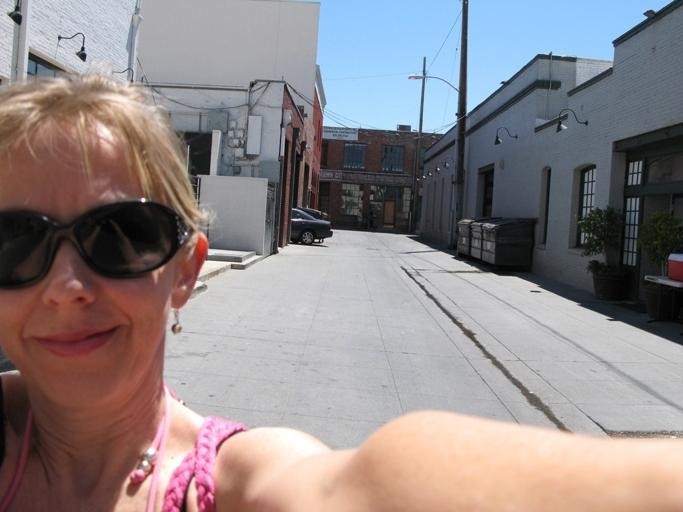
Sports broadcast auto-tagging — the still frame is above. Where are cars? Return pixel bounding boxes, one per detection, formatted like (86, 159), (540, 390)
(290, 208), (332, 244)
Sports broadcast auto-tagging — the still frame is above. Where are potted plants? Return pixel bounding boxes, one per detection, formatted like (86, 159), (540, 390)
(574, 205), (683, 321)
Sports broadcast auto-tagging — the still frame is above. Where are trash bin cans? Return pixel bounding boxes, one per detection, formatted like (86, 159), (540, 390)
(455, 215), (538, 268)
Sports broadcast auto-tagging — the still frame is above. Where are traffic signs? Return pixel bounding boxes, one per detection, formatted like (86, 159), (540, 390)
(407, 75), (458, 248)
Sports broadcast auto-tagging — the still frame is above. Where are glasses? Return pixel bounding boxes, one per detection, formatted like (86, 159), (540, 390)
(1, 199), (190, 290)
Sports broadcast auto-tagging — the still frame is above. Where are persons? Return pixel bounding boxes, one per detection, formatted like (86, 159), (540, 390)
(0, 72), (683, 512)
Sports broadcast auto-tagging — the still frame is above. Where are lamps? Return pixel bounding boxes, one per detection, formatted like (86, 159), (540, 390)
(556, 108), (588, 132)
(494, 126), (518, 145)
(59, 32), (87, 62)
(8, 0), (22, 25)
